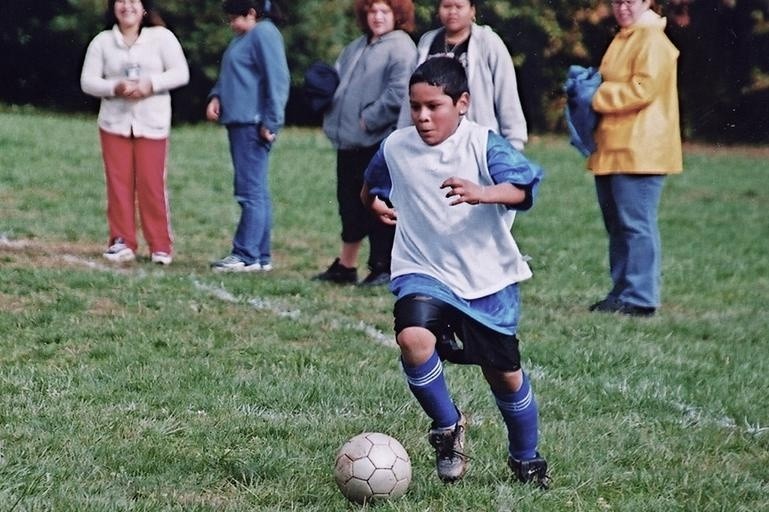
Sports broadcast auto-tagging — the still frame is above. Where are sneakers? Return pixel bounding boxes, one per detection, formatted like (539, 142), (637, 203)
(311, 257), (358, 284)
(507, 454), (551, 490)
(257, 262), (271, 271)
(357, 268), (391, 287)
(151, 251), (172, 265)
(103, 240), (135, 262)
(591, 297), (615, 310)
(428, 406), (467, 480)
(604, 300), (654, 316)
(212, 255), (261, 273)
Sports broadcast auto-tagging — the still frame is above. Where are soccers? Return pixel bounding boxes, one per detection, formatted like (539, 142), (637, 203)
(333, 432), (412, 507)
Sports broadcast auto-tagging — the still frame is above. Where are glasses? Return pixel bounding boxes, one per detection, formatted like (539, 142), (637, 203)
(610, 0), (638, 6)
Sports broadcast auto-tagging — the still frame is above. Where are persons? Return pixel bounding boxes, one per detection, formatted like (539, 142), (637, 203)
(391, 0), (527, 150)
(311, 1), (420, 288)
(79, 0), (190, 261)
(360, 55), (555, 485)
(206, 1), (291, 271)
(578, 0), (680, 314)
